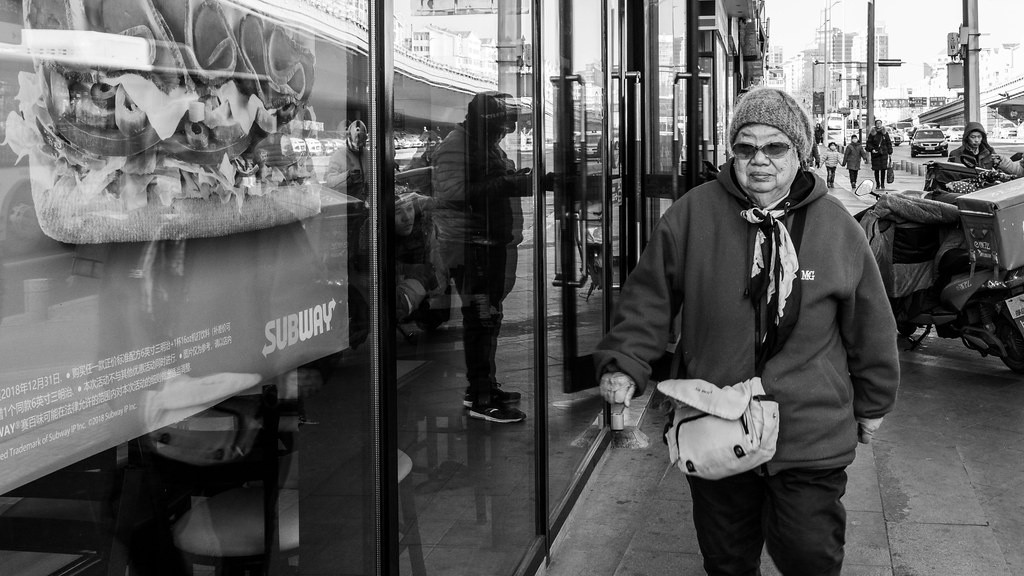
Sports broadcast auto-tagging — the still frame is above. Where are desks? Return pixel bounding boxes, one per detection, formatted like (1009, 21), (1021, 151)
(396, 359), (437, 575)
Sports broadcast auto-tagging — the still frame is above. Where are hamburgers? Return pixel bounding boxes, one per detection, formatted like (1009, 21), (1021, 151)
(4, 1), (322, 242)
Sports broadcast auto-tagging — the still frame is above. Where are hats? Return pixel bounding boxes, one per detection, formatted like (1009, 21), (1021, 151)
(730, 88), (813, 168)
(470, 94), (518, 123)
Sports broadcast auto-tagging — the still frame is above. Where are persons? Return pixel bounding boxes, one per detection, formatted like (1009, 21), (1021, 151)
(948, 122), (997, 170)
(816, 124), (825, 146)
(595, 88), (900, 576)
(807, 142), (820, 168)
(325, 91), (565, 421)
(842, 135), (868, 188)
(706, 165), (723, 180)
(820, 142), (843, 188)
(991, 154), (1024, 177)
(865, 120), (893, 189)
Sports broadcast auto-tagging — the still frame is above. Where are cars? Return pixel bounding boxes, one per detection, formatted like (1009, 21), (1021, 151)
(909, 128), (951, 157)
(846, 122), (966, 145)
(573, 130), (602, 159)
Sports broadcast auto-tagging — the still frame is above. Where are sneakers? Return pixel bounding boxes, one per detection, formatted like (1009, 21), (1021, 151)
(469, 402), (526, 423)
(461, 386), (521, 409)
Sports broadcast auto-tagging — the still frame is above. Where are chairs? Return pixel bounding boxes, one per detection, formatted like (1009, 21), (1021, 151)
(0, 388), (413, 576)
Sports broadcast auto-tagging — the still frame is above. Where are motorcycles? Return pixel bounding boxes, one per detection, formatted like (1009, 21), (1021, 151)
(850, 179), (1024, 376)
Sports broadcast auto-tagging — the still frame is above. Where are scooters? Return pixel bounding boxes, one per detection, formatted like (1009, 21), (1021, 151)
(569, 208), (605, 301)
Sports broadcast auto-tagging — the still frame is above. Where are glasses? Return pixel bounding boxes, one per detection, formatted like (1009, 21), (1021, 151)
(490, 124), (516, 136)
(731, 142), (795, 159)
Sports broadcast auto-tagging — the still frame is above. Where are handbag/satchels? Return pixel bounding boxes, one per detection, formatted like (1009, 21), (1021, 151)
(872, 147), (883, 159)
(656, 377), (780, 479)
(887, 157), (894, 184)
